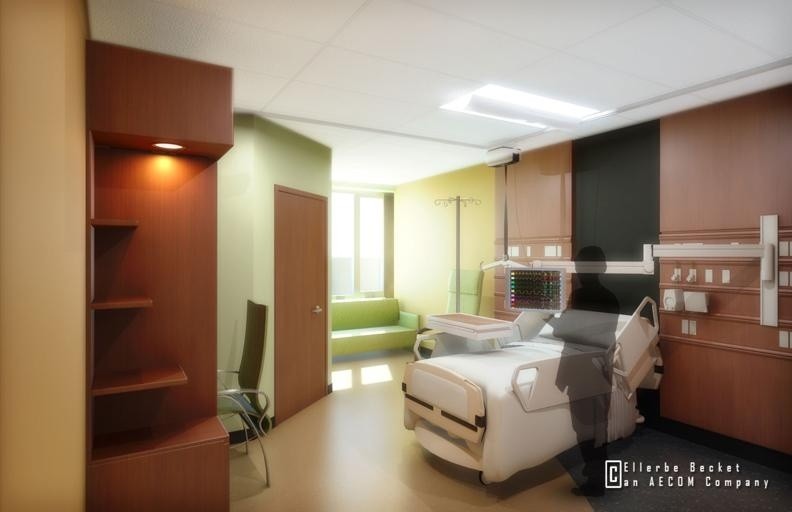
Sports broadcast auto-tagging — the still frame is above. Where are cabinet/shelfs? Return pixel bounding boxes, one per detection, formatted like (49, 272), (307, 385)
(86, 40), (233, 512)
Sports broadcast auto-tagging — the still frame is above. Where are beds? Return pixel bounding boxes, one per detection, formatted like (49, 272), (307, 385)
(402, 297), (663, 486)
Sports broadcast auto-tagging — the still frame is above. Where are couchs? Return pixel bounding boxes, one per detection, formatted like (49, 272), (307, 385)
(331, 297), (421, 357)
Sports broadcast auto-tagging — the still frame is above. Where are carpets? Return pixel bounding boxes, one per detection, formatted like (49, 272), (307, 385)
(557, 424), (792, 512)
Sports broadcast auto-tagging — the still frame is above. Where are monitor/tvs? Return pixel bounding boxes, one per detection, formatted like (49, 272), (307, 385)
(508, 268), (564, 312)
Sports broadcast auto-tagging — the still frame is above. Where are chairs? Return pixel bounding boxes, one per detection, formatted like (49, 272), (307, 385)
(217, 300), (272, 487)
(415, 269), (485, 360)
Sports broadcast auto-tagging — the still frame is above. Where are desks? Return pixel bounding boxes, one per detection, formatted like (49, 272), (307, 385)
(424, 313), (513, 340)
